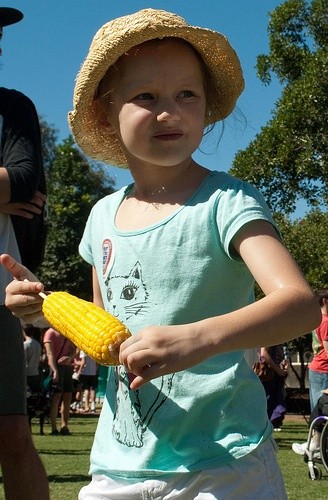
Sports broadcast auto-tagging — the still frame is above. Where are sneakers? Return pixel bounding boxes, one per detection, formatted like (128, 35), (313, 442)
(292, 438), (321, 459)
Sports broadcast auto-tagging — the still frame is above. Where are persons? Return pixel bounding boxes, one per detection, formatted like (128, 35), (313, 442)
(252, 343), (288, 427)
(307, 290), (328, 436)
(292, 389), (328, 459)
(28, 327), (97, 435)
(43, 324), (76, 435)
(0, 6), (51, 500)
(0, 8), (322, 500)
(20, 323), (42, 395)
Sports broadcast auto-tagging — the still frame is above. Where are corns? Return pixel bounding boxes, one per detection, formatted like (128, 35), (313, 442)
(42, 291), (132, 366)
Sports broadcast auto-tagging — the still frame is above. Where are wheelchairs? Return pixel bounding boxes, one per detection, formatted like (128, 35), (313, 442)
(303, 415), (328, 480)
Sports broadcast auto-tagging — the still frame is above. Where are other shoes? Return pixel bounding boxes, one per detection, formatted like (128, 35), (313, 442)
(71, 401), (96, 414)
(60, 426), (70, 435)
(49, 430), (58, 435)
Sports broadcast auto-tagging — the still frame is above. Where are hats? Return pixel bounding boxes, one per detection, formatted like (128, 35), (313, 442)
(68, 8), (245, 169)
(0, 7), (24, 27)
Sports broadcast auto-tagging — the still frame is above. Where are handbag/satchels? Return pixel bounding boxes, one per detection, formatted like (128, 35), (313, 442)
(42, 376), (60, 392)
